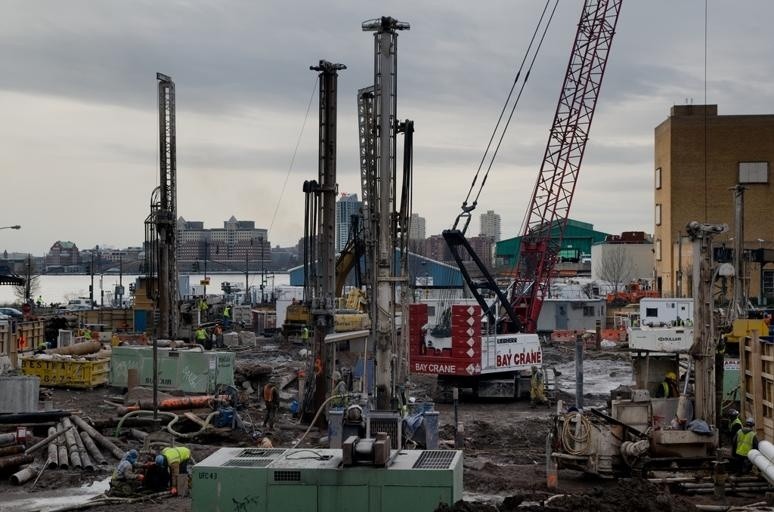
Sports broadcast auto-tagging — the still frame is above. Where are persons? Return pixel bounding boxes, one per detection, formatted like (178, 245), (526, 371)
(727, 408), (758, 477)
(262, 377), (280, 432)
(195, 296), (232, 351)
(301, 324), (308, 346)
(329, 371), (349, 407)
(105, 446), (192, 498)
(33, 295), (119, 356)
(529, 366), (551, 409)
(654, 372), (680, 398)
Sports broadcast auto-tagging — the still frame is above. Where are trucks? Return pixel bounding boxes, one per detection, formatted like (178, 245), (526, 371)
(607, 278), (662, 306)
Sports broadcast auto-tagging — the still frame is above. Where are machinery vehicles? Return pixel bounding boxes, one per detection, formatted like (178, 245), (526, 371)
(723, 182), (774, 360)
(545, 220), (730, 495)
(406, 0), (626, 399)
(551, 316), (633, 352)
(281, 209), (409, 349)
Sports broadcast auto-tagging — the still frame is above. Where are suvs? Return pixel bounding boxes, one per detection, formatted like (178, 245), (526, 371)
(58, 299), (92, 310)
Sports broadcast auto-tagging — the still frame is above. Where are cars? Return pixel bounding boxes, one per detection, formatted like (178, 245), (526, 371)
(0, 307), (23, 320)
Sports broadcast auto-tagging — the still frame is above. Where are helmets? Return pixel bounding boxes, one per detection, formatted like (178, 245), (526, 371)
(253, 431), (262, 441)
(746, 418), (754, 425)
(665, 372), (676, 381)
(728, 409), (739, 416)
(155, 455), (167, 469)
(126, 449), (139, 464)
(331, 371), (340, 380)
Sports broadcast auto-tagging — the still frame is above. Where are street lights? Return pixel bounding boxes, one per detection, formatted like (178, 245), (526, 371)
(81, 248), (101, 306)
(110, 252), (126, 310)
(0, 225), (21, 230)
